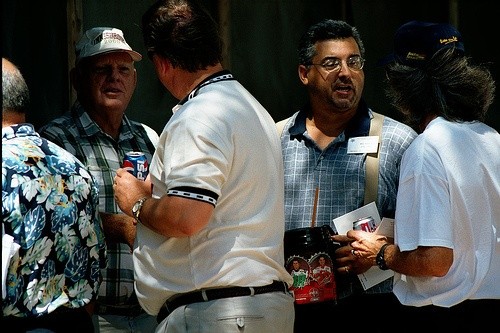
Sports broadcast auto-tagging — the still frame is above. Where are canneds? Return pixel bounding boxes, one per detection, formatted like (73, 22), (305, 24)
(123, 151), (149, 182)
(352, 216), (375, 232)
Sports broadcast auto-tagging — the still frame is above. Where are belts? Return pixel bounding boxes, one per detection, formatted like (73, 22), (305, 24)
(158, 282), (289, 326)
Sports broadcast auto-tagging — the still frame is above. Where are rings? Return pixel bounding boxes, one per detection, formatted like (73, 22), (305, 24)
(351, 250), (354, 254)
(346, 265), (348, 271)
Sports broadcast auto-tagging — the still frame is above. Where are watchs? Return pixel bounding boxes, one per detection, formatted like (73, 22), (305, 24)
(376, 243), (388, 271)
(132, 195), (149, 223)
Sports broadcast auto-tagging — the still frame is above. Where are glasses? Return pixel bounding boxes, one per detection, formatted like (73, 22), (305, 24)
(307, 57), (367, 72)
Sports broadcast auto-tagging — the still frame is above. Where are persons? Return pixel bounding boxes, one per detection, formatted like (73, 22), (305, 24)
(289, 260), (307, 283)
(311, 255), (330, 278)
(276, 21), (431, 333)
(113, 0), (296, 333)
(2, 59), (114, 333)
(40, 27), (162, 333)
(331, 31), (500, 333)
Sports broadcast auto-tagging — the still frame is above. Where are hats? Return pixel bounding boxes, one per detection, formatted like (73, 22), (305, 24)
(368, 20), (466, 71)
(74, 27), (142, 62)
